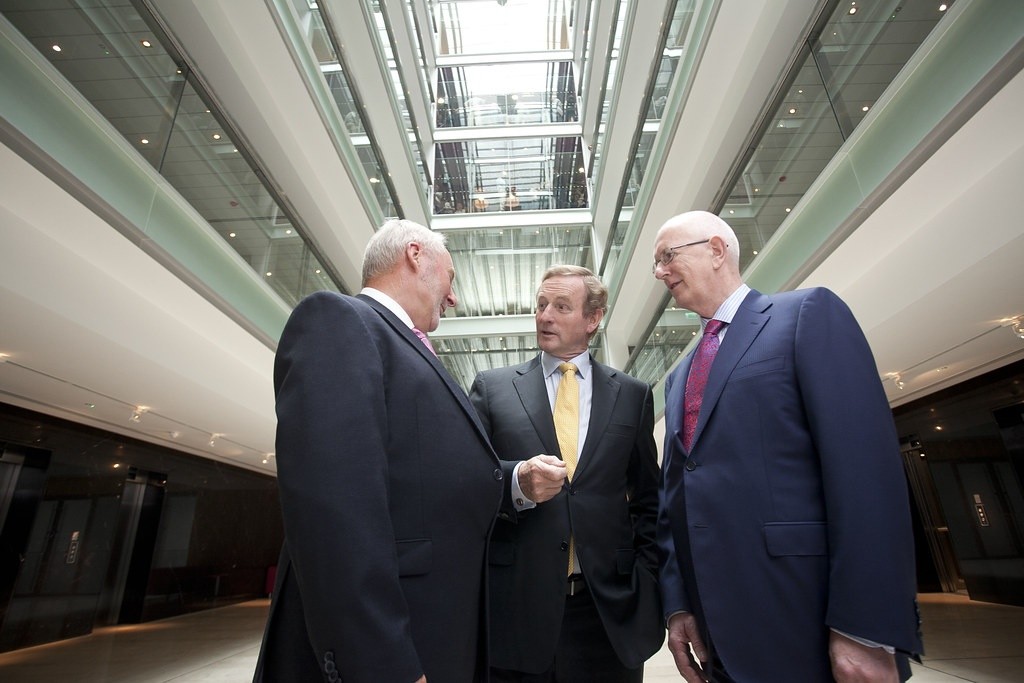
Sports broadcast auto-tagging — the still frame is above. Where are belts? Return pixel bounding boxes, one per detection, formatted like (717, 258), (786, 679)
(563, 580), (587, 597)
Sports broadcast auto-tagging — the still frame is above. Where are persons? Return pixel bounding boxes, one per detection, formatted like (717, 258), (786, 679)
(468, 265), (665, 683)
(434, 173), (467, 214)
(652, 211), (925, 683)
(254, 217), (504, 683)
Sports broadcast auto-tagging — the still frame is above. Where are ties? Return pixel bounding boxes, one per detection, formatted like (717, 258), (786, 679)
(411, 327), (438, 358)
(552, 362), (579, 578)
(679, 319), (726, 454)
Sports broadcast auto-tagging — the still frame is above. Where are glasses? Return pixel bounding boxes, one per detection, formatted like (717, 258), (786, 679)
(652, 239), (728, 276)
